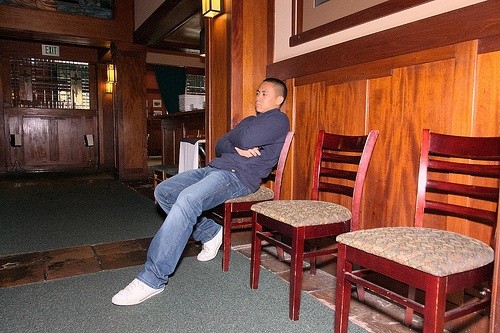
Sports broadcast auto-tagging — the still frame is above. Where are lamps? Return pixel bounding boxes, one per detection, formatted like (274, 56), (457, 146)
(106, 78), (113, 94)
(105, 62), (115, 83)
(202, 0), (224, 20)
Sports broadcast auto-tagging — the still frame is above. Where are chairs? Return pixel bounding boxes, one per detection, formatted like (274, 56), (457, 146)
(335, 128), (500, 333)
(202, 131), (294, 272)
(152, 163), (179, 204)
(250, 129), (380, 321)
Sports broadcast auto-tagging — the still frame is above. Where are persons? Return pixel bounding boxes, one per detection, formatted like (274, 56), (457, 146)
(112, 78), (290, 305)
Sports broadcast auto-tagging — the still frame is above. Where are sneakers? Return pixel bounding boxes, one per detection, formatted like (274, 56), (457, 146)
(111, 278), (165, 306)
(197, 227), (222, 261)
